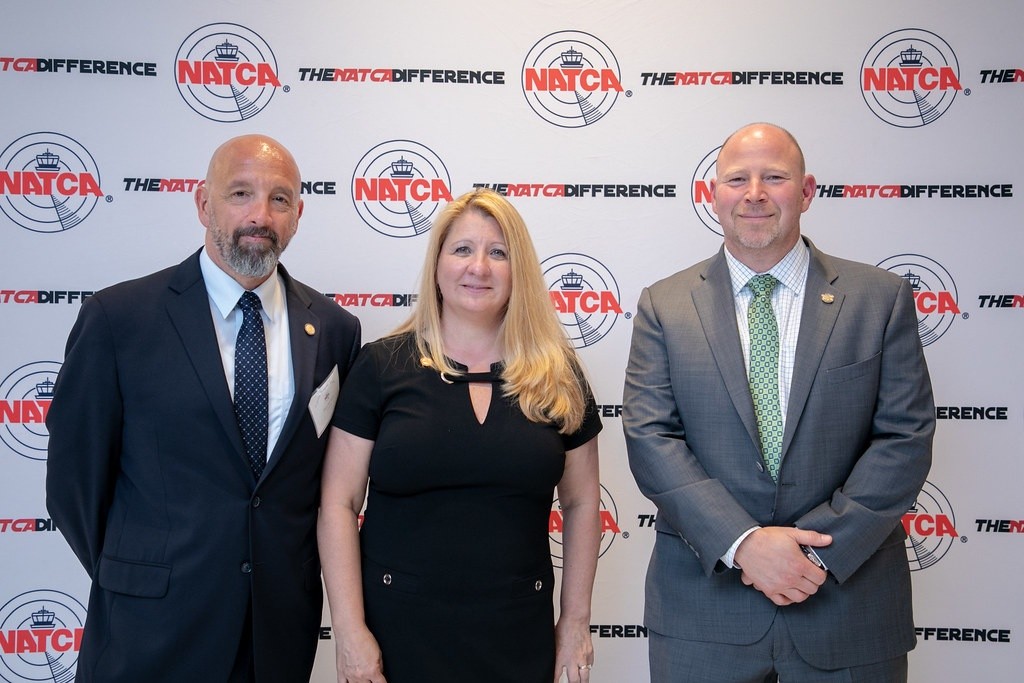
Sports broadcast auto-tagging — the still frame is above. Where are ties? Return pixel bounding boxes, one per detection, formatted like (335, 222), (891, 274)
(744, 275), (783, 483)
(233, 292), (269, 482)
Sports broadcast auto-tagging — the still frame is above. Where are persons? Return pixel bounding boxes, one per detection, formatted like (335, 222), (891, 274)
(45, 133), (362, 683)
(318, 188), (604, 683)
(620, 123), (937, 683)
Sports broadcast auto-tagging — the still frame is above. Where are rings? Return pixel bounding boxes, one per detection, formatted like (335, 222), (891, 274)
(579, 664), (592, 670)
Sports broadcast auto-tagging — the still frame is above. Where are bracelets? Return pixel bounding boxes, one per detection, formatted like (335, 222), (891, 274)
(800, 545), (825, 570)
